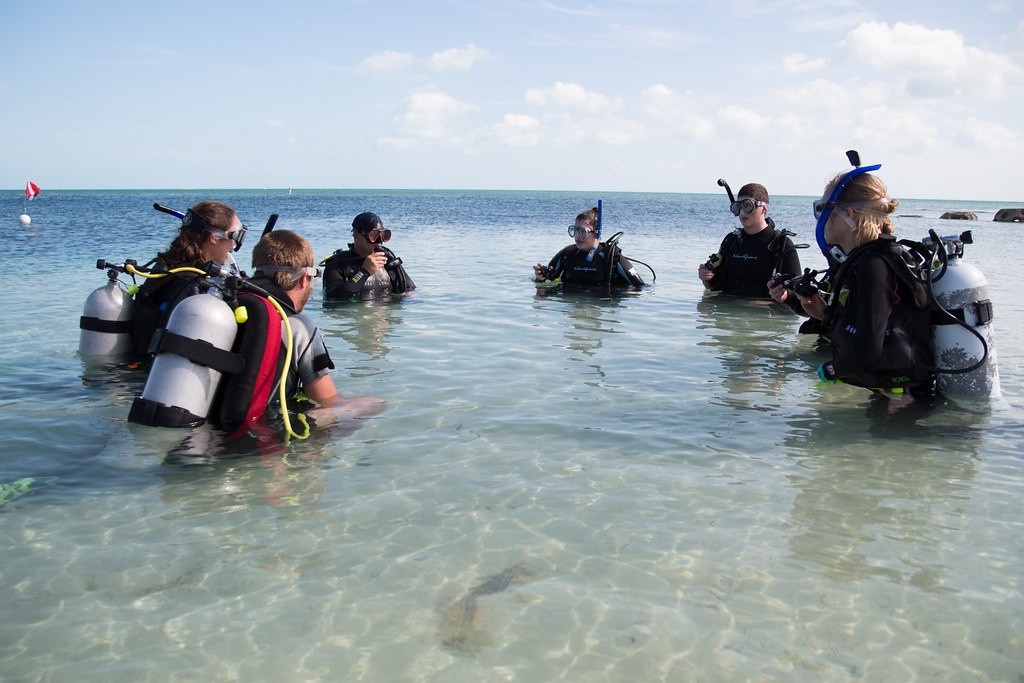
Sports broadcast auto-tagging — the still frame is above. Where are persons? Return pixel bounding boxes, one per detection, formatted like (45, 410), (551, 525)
(322, 211), (416, 309)
(133, 200), (241, 364)
(698, 183), (802, 315)
(206, 229), (386, 450)
(767, 172), (933, 418)
(533, 206), (645, 297)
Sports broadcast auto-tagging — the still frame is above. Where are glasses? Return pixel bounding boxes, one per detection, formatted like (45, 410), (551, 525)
(568, 223), (592, 240)
(813, 199), (825, 220)
(367, 229), (391, 243)
(730, 198), (759, 217)
(232, 223), (249, 252)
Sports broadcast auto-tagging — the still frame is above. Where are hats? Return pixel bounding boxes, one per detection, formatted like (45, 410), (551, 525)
(352, 212), (383, 243)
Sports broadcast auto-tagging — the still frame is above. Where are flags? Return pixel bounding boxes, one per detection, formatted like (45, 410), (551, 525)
(25, 181), (40, 201)
